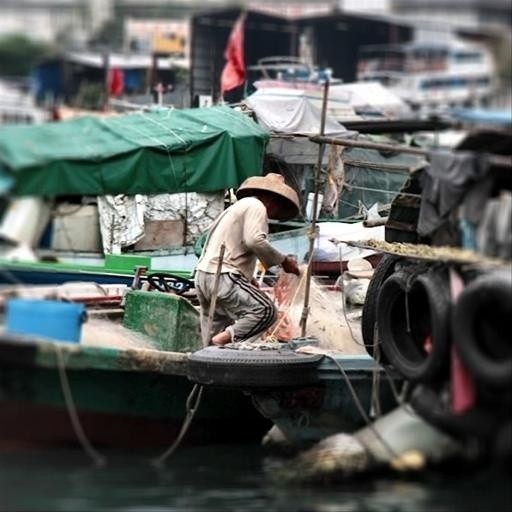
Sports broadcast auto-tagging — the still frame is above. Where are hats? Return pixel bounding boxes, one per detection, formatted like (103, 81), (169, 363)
(236, 173), (300, 220)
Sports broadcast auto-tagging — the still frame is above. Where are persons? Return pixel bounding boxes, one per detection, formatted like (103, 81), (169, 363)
(194, 173), (299, 348)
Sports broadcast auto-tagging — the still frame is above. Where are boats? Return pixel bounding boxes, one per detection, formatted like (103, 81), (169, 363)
(1, 36), (511, 438)
